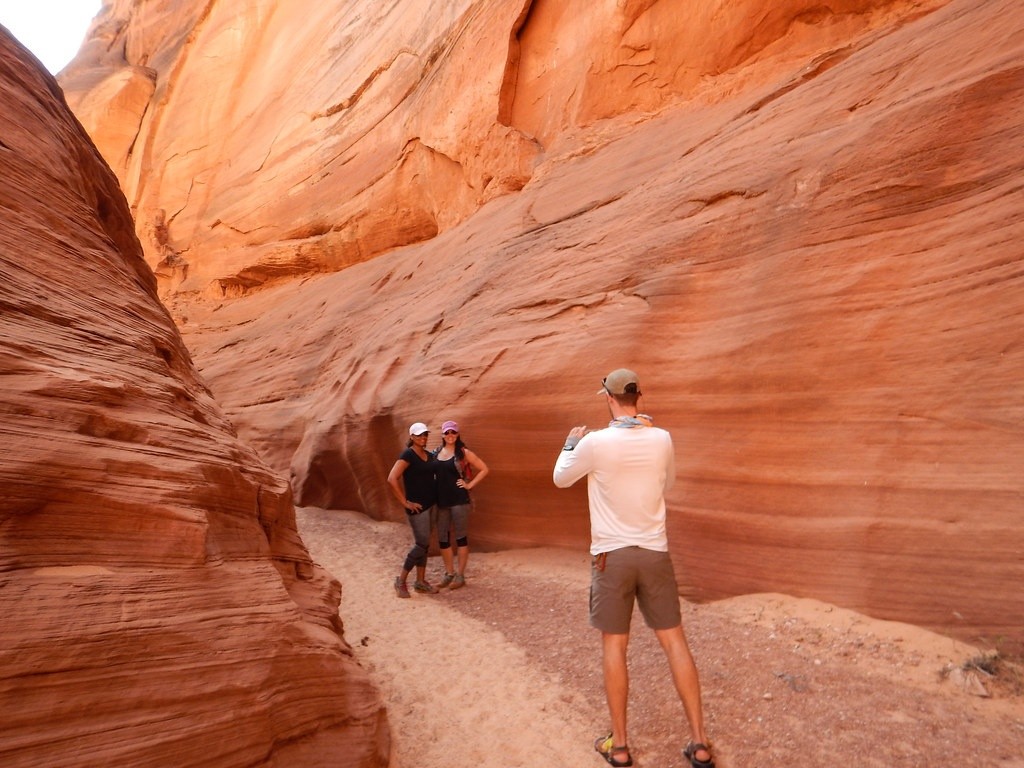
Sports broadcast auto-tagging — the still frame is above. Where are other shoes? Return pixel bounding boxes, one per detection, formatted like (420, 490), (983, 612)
(449, 574), (464, 589)
(438, 572), (454, 587)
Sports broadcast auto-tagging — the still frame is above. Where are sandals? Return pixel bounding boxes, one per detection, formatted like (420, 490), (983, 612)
(685, 740), (715, 768)
(595, 732), (632, 767)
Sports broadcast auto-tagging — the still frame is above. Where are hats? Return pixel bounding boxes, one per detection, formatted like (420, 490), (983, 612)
(409, 423), (431, 436)
(441, 421), (458, 433)
(597, 368), (641, 395)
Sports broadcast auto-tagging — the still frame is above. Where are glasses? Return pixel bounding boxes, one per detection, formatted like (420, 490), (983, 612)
(602, 377), (613, 397)
(444, 430), (456, 435)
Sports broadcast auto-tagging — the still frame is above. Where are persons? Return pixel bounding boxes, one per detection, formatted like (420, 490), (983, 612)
(553, 368), (717, 768)
(388, 423), (438, 598)
(431, 421), (489, 588)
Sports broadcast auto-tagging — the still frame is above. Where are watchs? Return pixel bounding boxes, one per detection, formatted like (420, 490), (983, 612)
(564, 445), (573, 451)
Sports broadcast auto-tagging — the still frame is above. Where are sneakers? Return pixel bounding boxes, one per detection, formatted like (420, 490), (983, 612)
(414, 580), (438, 594)
(394, 576), (410, 598)
